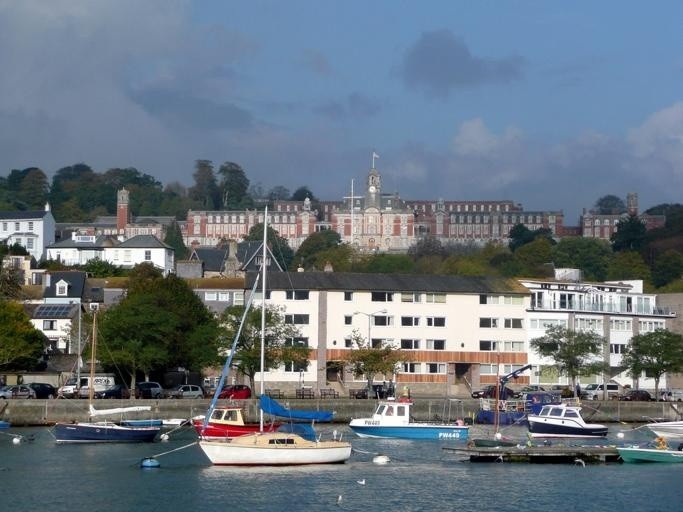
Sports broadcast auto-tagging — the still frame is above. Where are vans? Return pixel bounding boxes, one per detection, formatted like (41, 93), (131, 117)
(580, 382), (619, 400)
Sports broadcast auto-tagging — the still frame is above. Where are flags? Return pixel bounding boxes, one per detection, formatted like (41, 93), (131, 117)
(374, 152), (381, 159)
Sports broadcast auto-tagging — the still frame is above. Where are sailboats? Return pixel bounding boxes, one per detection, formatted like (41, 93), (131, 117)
(53, 306), (161, 444)
(198, 205), (352, 465)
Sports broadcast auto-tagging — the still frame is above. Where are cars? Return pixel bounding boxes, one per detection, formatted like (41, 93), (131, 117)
(165, 384), (204, 399)
(95, 385), (139, 399)
(213, 385), (251, 399)
(357, 385), (393, 399)
(519, 384), (580, 398)
(471, 384), (514, 398)
(128, 381), (164, 399)
(0, 382), (54, 399)
(619, 389), (651, 402)
(659, 387), (683, 403)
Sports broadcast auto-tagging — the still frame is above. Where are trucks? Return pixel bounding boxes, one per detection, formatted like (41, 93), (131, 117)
(56, 372), (115, 398)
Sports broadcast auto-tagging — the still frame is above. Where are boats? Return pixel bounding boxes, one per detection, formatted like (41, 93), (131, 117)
(349, 400), (469, 441)
(616, 446), (683, 463)
(189, 403), (312, 438)
(477, 393), (582, 424)
(473, 438), (517, 447)
(618, 420), (683, 441)
(527, 404), (608, 436)
(123, 418), (163, 427)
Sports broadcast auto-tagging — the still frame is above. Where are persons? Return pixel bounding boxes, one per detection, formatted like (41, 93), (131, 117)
(577, 382), (581, 397)
(455, 416), (464, 426)
(660, 388), (673, 401)
(381, 379), (395, 400)
